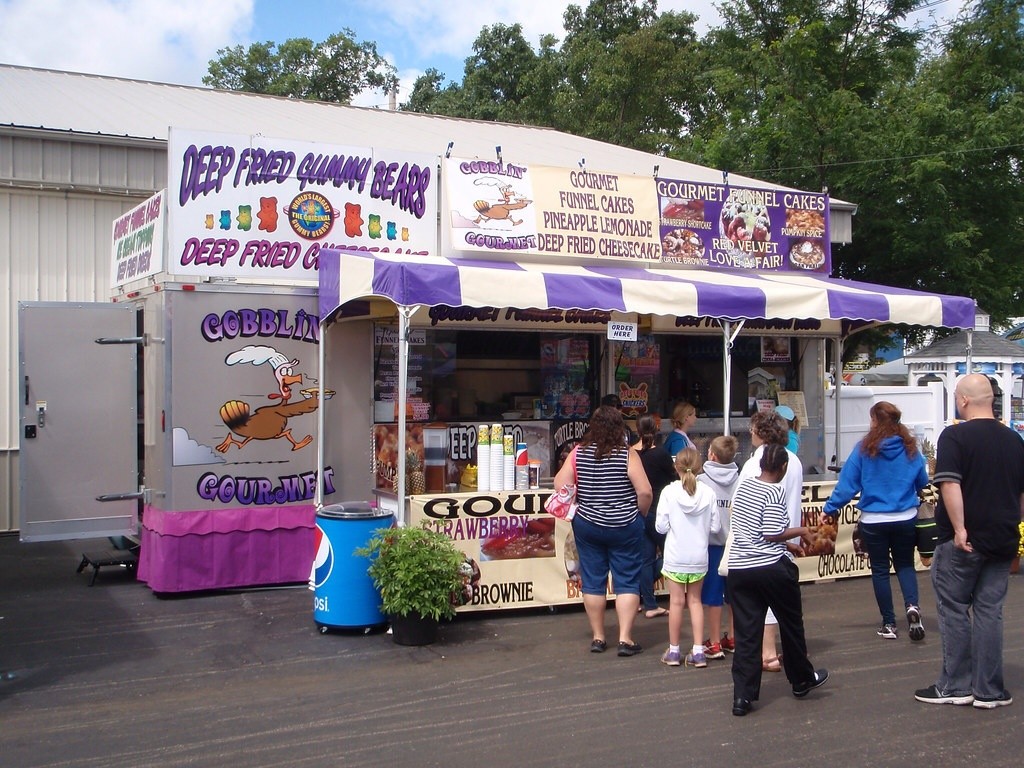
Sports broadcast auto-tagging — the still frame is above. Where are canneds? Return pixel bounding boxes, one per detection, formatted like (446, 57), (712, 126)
(532, 399), (541, 420)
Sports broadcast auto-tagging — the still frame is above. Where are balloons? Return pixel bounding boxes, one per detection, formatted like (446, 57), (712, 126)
(824, 372), (866, 386)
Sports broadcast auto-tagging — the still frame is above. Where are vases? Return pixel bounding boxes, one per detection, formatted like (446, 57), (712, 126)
(1010, 556), (1020, 573)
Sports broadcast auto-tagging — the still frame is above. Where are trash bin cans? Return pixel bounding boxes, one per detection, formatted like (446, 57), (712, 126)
(313, 499), (397, 635)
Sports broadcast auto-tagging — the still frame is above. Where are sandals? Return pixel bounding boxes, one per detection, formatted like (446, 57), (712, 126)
(762, 656), (782, 673)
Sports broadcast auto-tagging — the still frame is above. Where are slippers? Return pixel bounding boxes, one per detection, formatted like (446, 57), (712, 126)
(644, 607), (670, 619)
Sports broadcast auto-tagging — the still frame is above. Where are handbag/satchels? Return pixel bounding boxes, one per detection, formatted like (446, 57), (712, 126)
(914, 495), (939, 558)
(544, 444), (581, 521)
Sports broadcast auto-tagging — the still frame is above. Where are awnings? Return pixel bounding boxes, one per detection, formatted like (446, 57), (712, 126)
(314, 247), (975, 634)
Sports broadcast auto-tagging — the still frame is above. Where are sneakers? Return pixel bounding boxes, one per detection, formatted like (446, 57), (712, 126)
(703, 638), (726, 660)
(617, 641), (646, 657)
(905, 604), (926, 641)
(973, 688), (1013, 710)
(684, 648), (707, 668)
(719, 632), (736, 653)
(876, 622), (897, 639)
(590, 639), (608, 653)
(660, 648), (682, 666)
(914, 683), (975, 705)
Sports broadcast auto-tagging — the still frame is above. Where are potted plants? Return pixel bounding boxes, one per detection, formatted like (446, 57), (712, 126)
(353, 516), (467, 645)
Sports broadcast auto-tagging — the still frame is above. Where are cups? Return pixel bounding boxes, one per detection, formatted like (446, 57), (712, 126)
(476, 423), (541, 490)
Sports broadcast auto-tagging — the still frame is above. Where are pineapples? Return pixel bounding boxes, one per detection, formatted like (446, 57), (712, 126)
(392, 451), (425, 496)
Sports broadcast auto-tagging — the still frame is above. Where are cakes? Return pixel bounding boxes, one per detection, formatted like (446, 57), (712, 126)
(661, 191), (825, 269)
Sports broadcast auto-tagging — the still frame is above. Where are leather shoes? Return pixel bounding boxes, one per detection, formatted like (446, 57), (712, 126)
(792, 668), (832, 697)
(732, 697), (753, 716)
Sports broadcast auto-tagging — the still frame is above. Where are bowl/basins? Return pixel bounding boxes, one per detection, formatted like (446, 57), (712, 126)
(502, 412), (522, 419)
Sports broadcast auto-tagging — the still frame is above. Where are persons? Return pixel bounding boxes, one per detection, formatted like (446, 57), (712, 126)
(718, 409), (802, 671)
(696, 436), (738, 659)
(821, 401), (929, 641)
(654, 448), (722, 667)
(774, 405), (802, 455)
(553, 393), (680, 657)
(664, 402), (696, 464)
(914, 373), (1024, 709)
(728, 445), (829, 716)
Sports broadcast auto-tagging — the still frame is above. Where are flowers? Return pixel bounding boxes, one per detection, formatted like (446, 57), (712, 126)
(1017, 521), (1024, 557)
